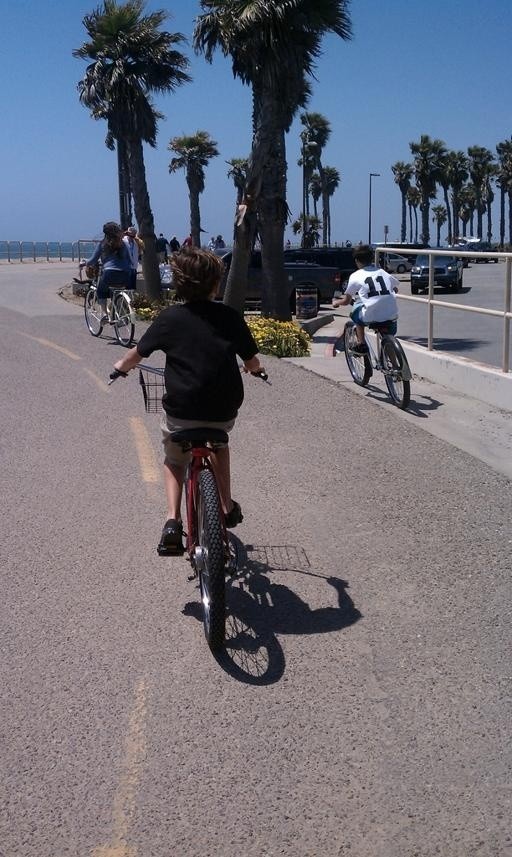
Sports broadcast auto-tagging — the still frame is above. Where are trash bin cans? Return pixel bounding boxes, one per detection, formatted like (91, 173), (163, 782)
(296, 285), (318, 318)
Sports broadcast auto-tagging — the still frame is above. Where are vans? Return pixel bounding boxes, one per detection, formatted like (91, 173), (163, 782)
(282, 248), (359, 296)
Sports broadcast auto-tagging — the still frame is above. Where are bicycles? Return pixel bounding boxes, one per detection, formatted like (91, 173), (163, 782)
(107, 363), (272, 645)
(332, 297), (412, 408)
(79, 263), (135, 347)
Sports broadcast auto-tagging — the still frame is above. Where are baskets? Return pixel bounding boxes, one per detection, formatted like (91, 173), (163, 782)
(140, 368), (166, 413)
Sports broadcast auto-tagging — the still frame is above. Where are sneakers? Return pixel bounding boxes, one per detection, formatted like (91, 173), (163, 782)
(101, 316), (108, 320)
(348, 344), (367, 354)
(225, 499), (241, 527)
(159, 519), (187, 545)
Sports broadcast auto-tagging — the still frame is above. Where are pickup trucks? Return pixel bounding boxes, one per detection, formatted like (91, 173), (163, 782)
(159, 247), (341, 313)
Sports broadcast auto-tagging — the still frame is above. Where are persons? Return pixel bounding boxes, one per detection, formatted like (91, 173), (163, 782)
(286, 239), (290, 248)
(122, 227), (145, 290)
(157, 234), (192, 264)
(112, 246), (260, 556)
(82, 221), (132, 325)
(333, 244), (400, 366)
(215, 235), (225, 249)
(208, 237), (215, 251)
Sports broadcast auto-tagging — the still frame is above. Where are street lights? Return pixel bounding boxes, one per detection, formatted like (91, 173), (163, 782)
(384, 225), (388, 271)
(369, 173), (380, 247)
(300, 131), (307, 247)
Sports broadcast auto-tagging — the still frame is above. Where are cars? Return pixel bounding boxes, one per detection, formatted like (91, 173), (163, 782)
(371, 242), (498, 295)
